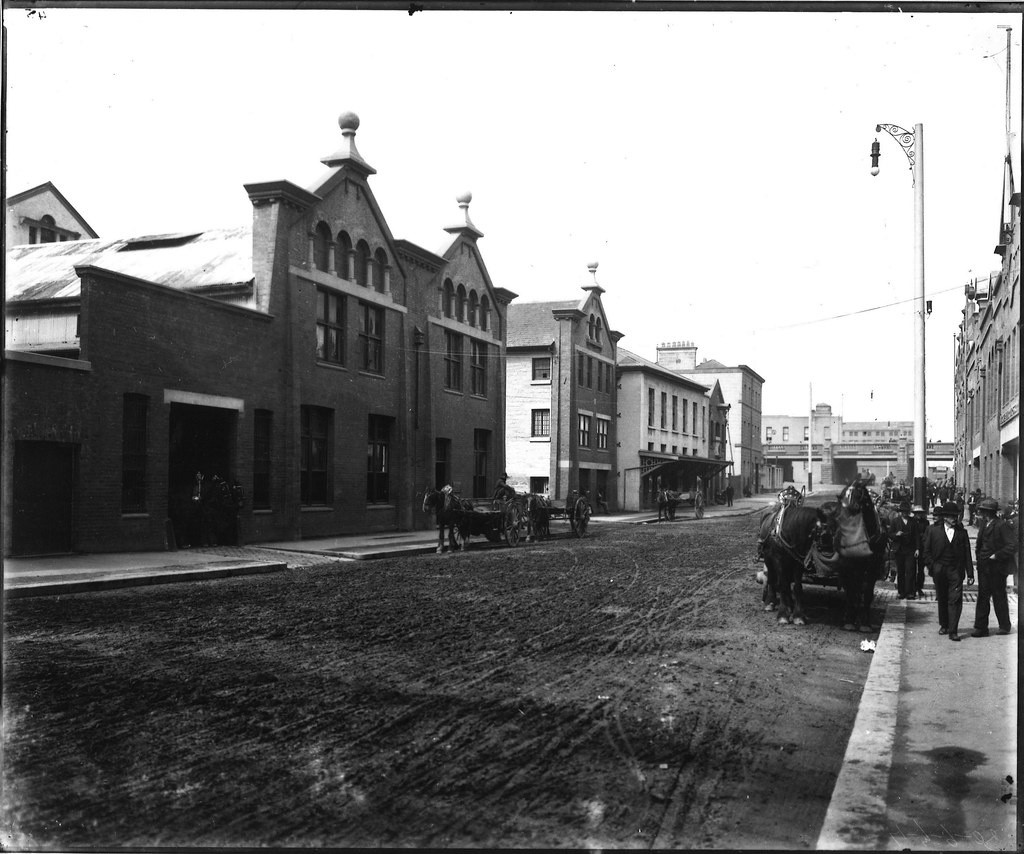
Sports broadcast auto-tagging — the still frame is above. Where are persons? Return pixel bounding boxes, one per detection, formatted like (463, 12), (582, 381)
(192, 471), (218, 547)
(726, 483), (734, 507)
(888, 500), (921, 600)
(857, 468), (1020, 594)
(492, 473), (515, 499)
(971, 497), (1020, 637)
(923, 502), (974, 641)
(598, 488), (610, 513)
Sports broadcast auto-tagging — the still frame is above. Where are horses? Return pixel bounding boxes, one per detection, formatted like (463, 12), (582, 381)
(521, 489), (550, 542)
(654, 482), (678, 519)
(759, 474), (887, 633)
(420, 485), (477, 554)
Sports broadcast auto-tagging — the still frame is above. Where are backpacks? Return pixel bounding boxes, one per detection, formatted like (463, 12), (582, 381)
(836, 507), (873, 557)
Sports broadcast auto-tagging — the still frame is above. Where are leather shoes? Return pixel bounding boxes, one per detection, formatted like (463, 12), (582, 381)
(971, 627), (1011, 637)
(897, 589), (926, 599)
(938, 625), (960, 641)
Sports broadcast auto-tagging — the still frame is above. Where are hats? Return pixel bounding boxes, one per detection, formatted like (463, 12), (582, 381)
(499, 473), (510, 478)
(930, 507), (944, 514)
(978, 499), (1002, 510)
(912, 504), (925, 512)
(896, 501), (911, 511)
(441, 485), (453, 494)
(942, 502), (961, 514)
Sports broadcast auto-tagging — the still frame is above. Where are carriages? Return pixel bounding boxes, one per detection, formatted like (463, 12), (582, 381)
(422, 487), (593, 555)
(746, 474), (900, 629)
(655, 487), (706, 523)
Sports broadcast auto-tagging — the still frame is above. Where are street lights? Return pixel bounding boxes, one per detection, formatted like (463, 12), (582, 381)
(869, 118), (927, 504)
